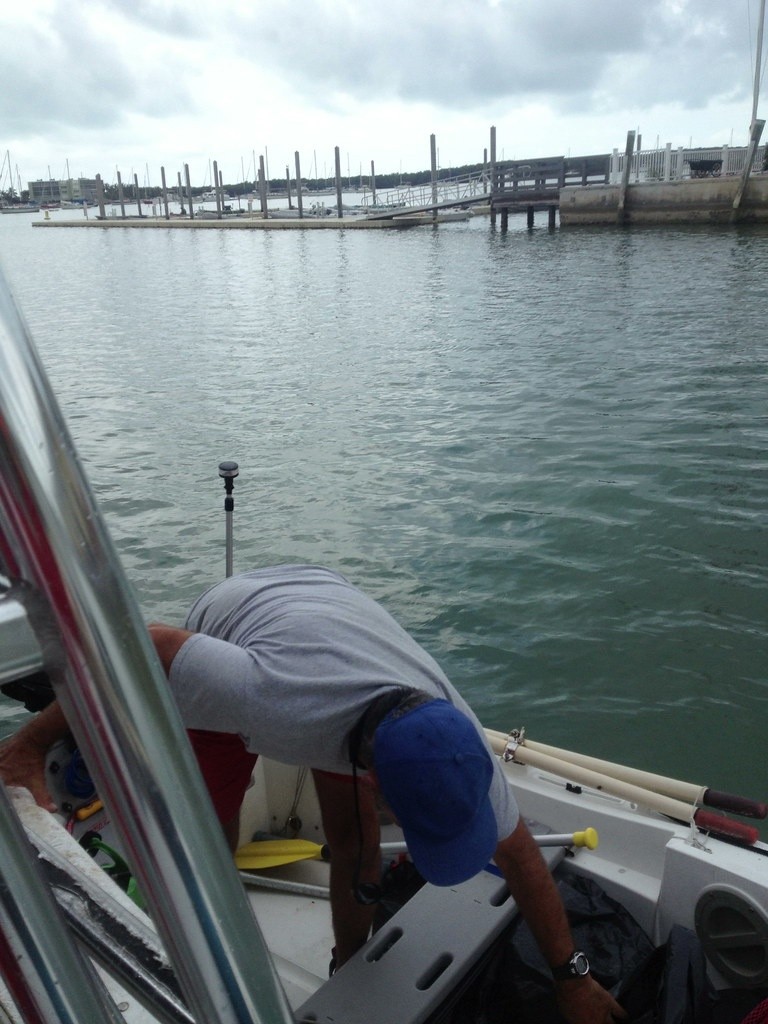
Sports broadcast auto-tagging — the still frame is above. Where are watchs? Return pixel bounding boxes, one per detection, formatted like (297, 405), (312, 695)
(551, 951), (590, 981)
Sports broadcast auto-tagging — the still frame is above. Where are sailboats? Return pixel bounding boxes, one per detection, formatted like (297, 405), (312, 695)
(201, 157), (231, 202)
(340, 153), (371, 194)
(242, 146), (332, 199)
(0, 150), (40, 213)
(392, 163), (413, 190)
(42, 158), (94, 210)
(109, 163), (163, 204)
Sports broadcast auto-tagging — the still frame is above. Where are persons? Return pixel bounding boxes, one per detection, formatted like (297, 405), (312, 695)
(0, 562), (626, 1024)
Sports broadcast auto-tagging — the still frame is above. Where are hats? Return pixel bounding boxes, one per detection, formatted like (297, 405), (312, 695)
(373, 692), (496, 887)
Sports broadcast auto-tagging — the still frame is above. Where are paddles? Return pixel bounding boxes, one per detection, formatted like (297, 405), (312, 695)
(232, 824), (599, 870)
